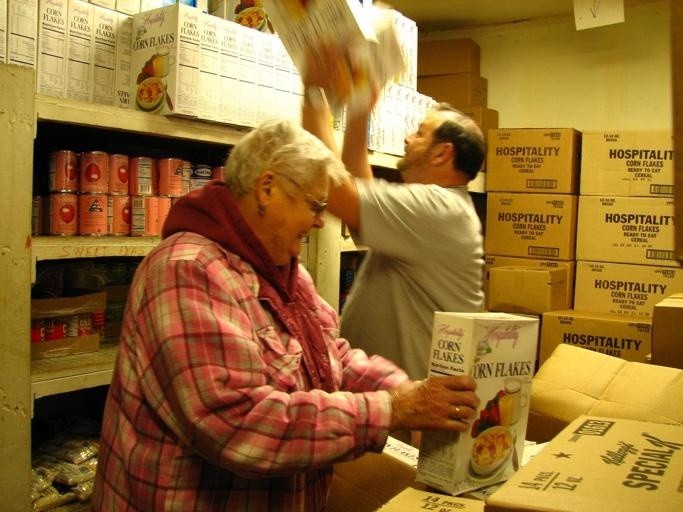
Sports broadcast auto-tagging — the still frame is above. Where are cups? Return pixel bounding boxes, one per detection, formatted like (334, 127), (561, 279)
(158, 45), (176, 77)
(504, 378), (528, 425)
(498, 397), (511, 428)
(152, 58), (160, 78)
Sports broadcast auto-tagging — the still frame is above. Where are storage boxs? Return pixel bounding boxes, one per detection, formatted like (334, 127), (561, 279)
(458, 106), (499, 130)
(483, 193), (574, 262)
(650, 304), (682, 372)
(379, 344), (682, 512)
(574, 197), (683, 267)
(417, 37), (481, 76)
(326, 422), (419, 507)
(416, 75), (487, 107)
(579, 131), (683, 199)
(485, 128), (580, 193)
(486, 255), (574, 311)
(573, 262), (683, 323)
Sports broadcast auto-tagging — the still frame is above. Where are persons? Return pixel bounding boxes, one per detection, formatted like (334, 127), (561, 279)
(299, 32), (488, 444)
(90, 121), (481, 512)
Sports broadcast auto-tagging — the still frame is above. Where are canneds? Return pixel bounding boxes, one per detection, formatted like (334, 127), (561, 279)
(31, 319), (46, 342)
(78, 195), (108, 235)
(46, 319), (67, 339)
(108, 195), (130, 235)
(192, 164), (211, 179)
(109, 154), (129, 195)
(48, 150), (78, 192)
(158, 197), (172, 236)
(159, 158), (183, 197)
(214, 166), (225, 180)
(79, 151), (109, 194)
(104, 301), (125, 337)
(183, 161), (191, 196)
(49, 193), (79, 234)
(32, 196), (43, 236)
(131, 197), (158, 236)
(193, 179), (210, 191)
(77, 258), (138, 290)
(92, 309), (107, 342)
(69, 314), (92, 338)
(131, 157), (158, 195)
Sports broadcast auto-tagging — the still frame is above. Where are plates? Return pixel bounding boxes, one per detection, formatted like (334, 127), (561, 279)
(259, 19), (267, 31)
(136, 90), (166, 115)
(466, 447), (515, 483)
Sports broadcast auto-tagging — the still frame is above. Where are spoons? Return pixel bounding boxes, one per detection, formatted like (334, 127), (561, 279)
(509, 429), (519, 471)
(162, 80), (173, 111)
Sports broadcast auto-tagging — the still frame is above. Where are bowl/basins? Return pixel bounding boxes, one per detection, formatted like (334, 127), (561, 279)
(137, 77), (163, 110)
(239, 7), (265, 28)
(471, 426), (512, 475)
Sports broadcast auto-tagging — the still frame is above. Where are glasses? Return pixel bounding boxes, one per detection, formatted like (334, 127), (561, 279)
(273, 171), (328, 212)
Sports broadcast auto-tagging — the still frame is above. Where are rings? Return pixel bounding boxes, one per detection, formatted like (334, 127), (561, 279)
(455, 405), (460, 420)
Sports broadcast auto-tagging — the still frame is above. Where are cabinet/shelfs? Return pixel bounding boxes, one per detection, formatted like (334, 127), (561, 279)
(0, 63), (340, 508)
